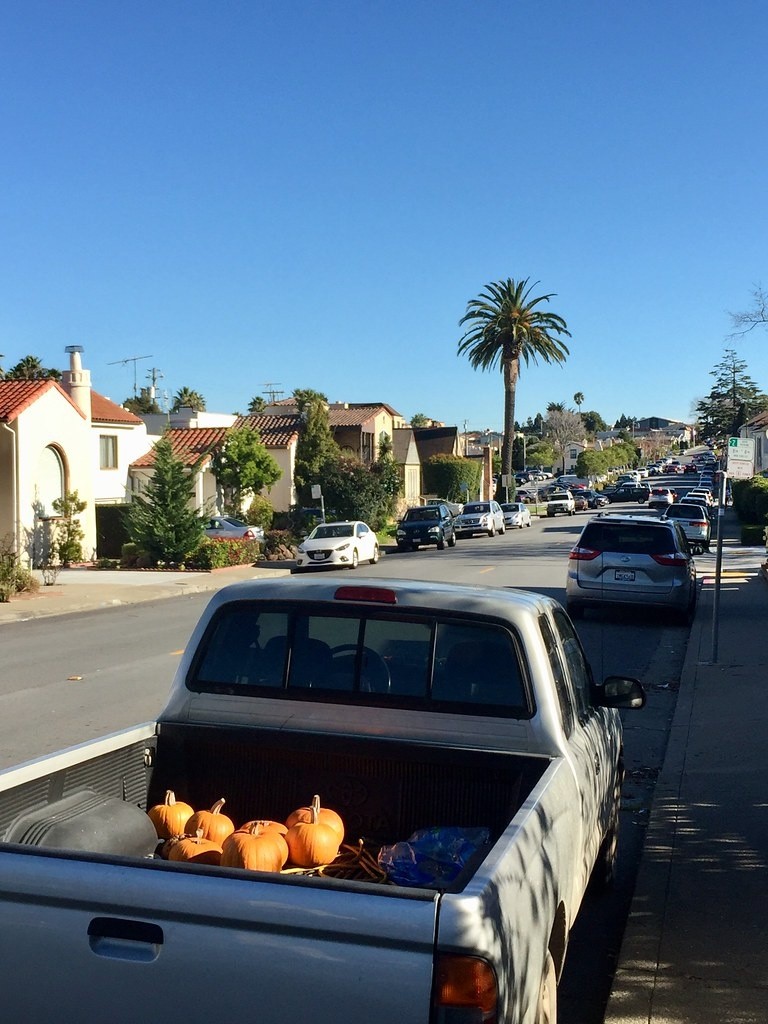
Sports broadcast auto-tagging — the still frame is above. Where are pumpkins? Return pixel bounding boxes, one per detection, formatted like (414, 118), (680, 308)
(286, 794), (344, 846)
(285, 808), (340, 866)
(169, 829), (222, 865)
(220, 824), (282, 873)
(147, 791), (194, 837)
(184, 798), (234, 845)
(239, 820), (288, 839)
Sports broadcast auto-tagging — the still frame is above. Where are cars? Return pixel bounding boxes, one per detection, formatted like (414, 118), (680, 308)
(492, 469), (611, 507)
(670, 489), (678, 503)
(672, 460), (682, 470)
(302, 508), (335, 522)
(684, 462), (698, 474)
(573, 496), (589, 511)
(667, 464), (678, 474)
(295, 520), (380, 571)
(199, 515), (268, 544)
(497, 502), (532, 528)
(648, 487), (674, 508)
(679, 450), (717, 519)
(662, 502), (712, 551)
(615, 457), (668, 492)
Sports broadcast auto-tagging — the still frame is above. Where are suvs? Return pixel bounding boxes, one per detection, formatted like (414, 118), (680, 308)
(546, 490), (576, 517)
(453, 501), (506, 537)
(600, 487), (650, 504)
(565, 510), (697, 627)
(395, 503), (457, 550)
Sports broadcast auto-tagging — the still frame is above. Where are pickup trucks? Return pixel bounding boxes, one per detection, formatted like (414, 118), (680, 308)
(1, 576), (645, 1024)
